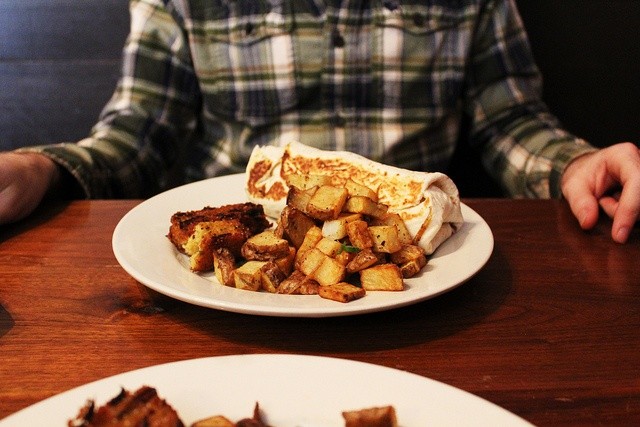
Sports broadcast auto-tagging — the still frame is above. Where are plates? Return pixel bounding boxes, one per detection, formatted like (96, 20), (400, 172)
(112, 160), (493, 317)
(2, 354), (539, 427)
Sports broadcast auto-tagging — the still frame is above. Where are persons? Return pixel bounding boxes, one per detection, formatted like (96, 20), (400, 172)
(1, 1), (636, 244)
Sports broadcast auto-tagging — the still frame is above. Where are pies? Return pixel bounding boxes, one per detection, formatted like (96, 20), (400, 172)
(244, 141), (465, 255)
